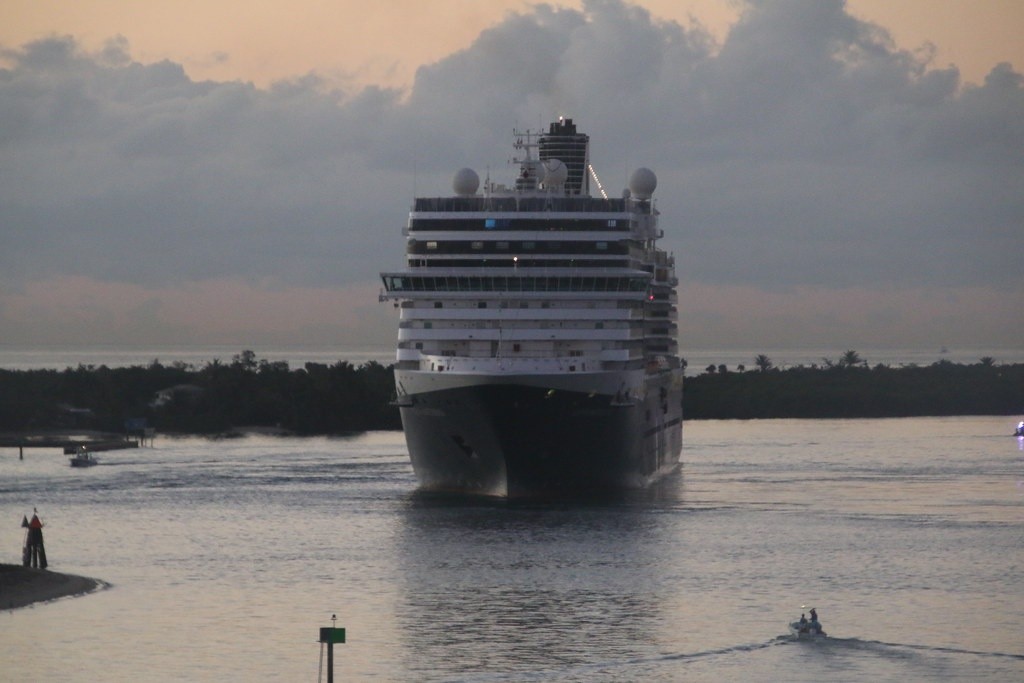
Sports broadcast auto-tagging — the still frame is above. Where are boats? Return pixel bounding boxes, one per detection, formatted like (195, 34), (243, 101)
(1013, 421), (1024, 451)
(68, 446), (98, 467)
(377, 116), (687, 508)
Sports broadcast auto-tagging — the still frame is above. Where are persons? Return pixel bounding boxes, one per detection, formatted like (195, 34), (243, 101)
(810, 608), (817, 622)
(800, 614), (807, 624)
